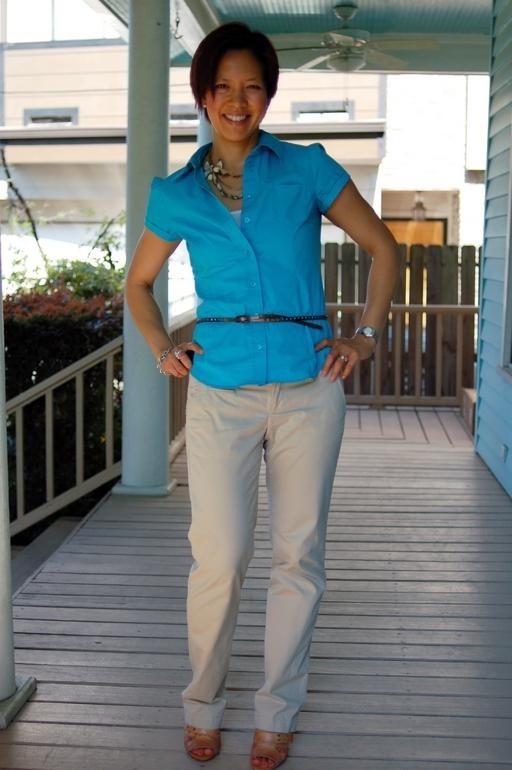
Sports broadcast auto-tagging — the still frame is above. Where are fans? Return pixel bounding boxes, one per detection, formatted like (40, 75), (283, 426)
(277, 2), (440, 80)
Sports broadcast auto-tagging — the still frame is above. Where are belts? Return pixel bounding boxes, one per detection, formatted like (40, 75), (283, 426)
(195, 311), (329, 330)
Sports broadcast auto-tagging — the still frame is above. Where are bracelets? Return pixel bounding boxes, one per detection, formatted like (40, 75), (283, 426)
(155, 345), (173, 375)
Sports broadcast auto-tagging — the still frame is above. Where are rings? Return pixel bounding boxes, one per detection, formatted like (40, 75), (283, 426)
(175, 349), (185, 358)
(338, 352), (349, 362)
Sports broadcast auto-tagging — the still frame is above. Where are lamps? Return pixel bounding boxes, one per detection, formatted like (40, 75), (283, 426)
(410, 192), (427, 218)
(325, 48), (373, 73)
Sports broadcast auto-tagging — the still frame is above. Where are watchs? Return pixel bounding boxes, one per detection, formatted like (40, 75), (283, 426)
(351, 325), (380, 345)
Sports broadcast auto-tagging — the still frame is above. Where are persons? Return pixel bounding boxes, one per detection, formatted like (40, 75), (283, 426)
(123, 21), (398, 770)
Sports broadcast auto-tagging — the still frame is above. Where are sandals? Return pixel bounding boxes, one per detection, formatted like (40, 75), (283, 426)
(250, 729), (291, 770)
(184, 723), (221, 761)
